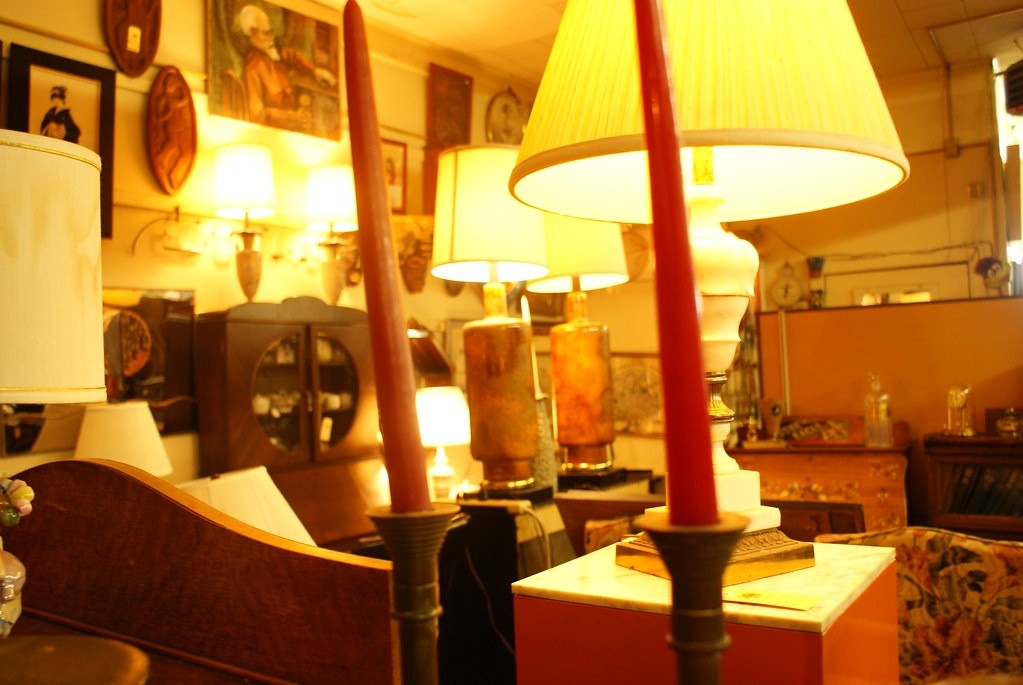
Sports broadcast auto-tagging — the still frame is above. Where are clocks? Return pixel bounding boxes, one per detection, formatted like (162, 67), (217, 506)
(770, 275), (801, 307)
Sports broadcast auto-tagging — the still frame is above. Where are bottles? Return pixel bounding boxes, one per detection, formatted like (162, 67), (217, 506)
(865, 373), (895, 449)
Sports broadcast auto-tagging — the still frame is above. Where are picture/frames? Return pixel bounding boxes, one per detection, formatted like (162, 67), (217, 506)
(424, 63), (474, 220)
(382, 137), (410, 216)
(6, 40), (115, 235)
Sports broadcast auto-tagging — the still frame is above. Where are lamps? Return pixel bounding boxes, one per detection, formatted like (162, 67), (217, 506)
(509, 0), (910, 593)
(178, 464), (317, 546)
(73, 403), (172, 482)
(0, 125), (109, 405)
(413, 386), (472, 498)
(523, 217), (653, 490)
(217, 150), (276, 303)
(435, 140), (552, 389)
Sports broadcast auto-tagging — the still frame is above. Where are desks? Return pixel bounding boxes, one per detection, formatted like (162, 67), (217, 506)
(0, 633), (149, 685)
(924, 432), (1023, 541)
(512, 532), (898, 685)
(727, 420), (907, 531)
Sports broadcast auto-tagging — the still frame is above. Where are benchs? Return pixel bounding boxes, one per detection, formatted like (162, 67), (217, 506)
(582, 516), (1023, 685)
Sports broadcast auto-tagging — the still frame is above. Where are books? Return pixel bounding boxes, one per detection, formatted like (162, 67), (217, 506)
(942, 465), (1023, 517)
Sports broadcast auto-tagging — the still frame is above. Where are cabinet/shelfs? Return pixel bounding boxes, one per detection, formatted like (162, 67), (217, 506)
(190, 293), (392, 549)
